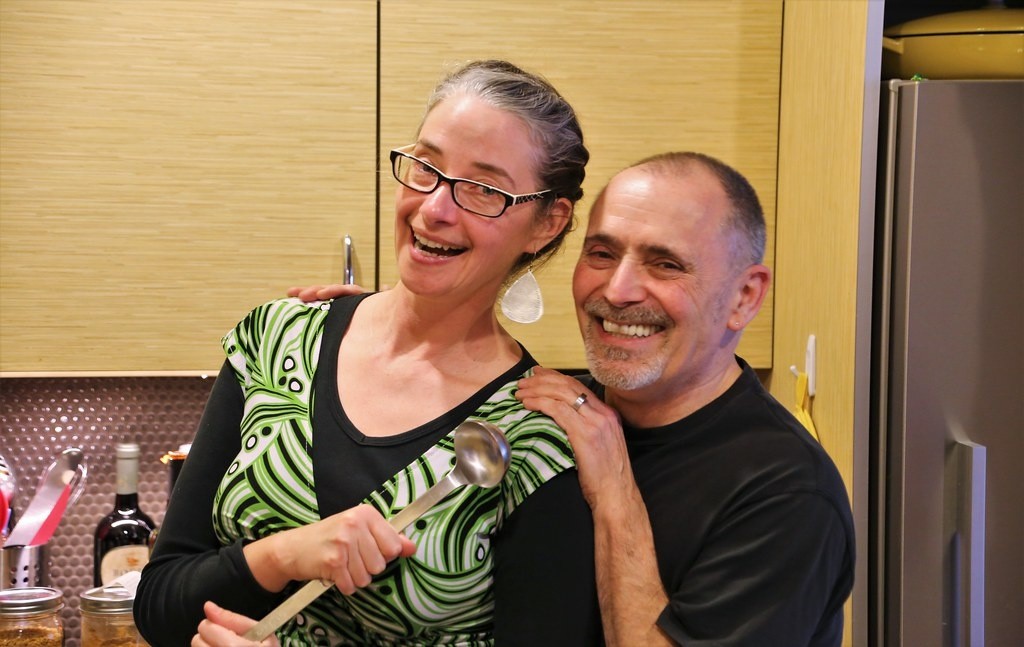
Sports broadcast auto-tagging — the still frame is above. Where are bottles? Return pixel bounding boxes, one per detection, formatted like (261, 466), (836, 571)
(94, 443), (158, 587)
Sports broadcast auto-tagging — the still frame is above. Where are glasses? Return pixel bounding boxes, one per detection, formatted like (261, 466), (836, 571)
(389, 145), (559, 218)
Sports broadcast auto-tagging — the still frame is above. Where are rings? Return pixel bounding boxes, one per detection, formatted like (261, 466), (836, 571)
(574, 393), (587, 411)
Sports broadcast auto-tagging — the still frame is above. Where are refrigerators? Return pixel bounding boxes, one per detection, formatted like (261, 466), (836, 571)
(870, 79), (1023, 644)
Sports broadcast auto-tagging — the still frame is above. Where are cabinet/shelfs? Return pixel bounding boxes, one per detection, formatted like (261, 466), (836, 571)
(0, 0), (786, 378)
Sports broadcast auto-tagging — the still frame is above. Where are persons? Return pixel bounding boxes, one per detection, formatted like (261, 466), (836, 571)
(492, 152), (856, 647)
(134, 61), (589, 647)
